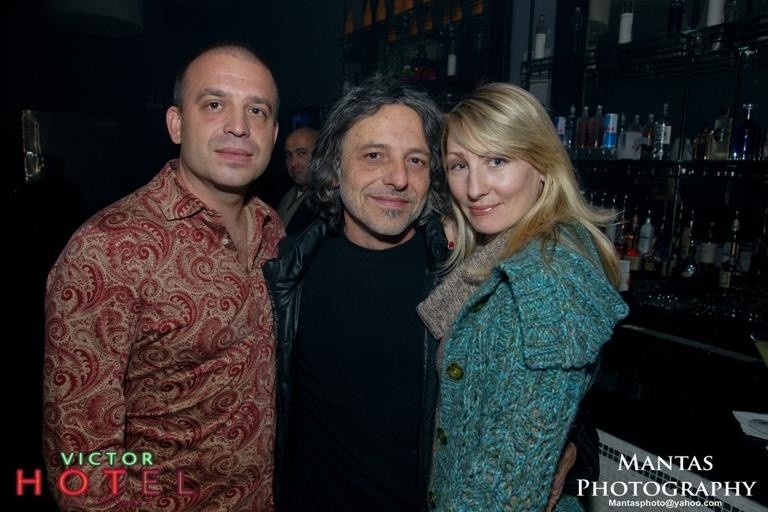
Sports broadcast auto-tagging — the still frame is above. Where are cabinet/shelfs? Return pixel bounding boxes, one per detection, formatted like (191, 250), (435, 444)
(519, 20), (768, 367)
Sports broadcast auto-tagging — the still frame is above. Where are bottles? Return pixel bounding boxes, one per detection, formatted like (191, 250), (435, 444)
(569, 6), (584, 51)
(617, 0), (637, 44)
(706, 0), (740, 28)
(534, 14), (547, 60)
(446, 33), (482, 79)
(550, 91), (766, 161)
(575, 192), (768, 297)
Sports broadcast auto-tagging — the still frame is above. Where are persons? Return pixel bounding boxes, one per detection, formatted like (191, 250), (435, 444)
(412, 75), (637, 512)
(275, 126), (321, 230)
(252, 67), (599, 511)
(40, 45), (289, 511)
